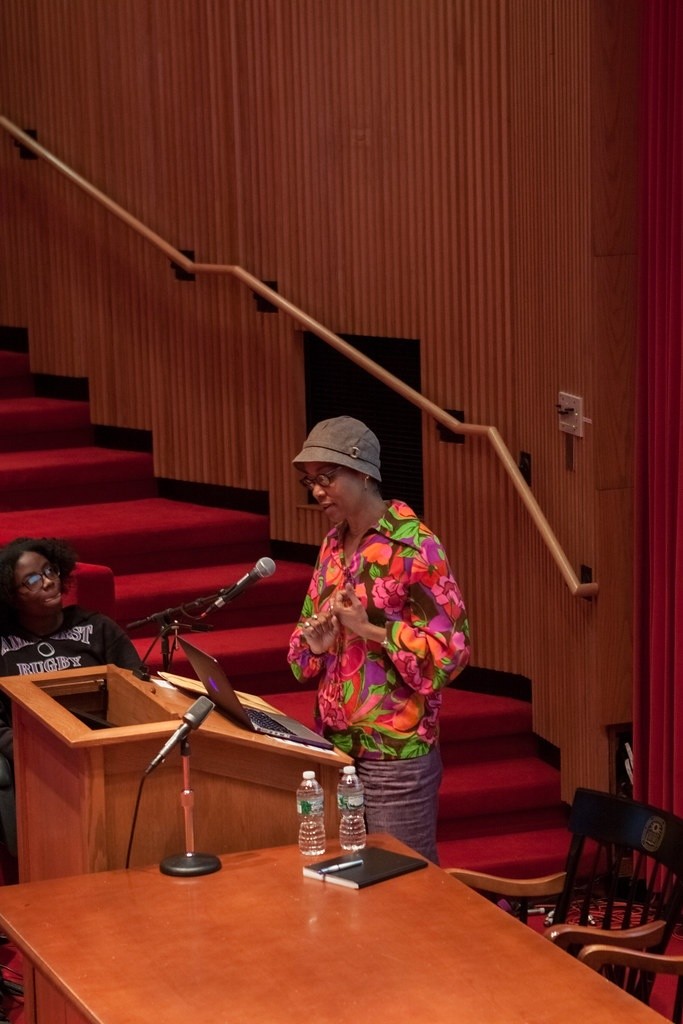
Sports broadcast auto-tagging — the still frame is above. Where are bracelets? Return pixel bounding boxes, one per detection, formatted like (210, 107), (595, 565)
(382, 630), (388, 648)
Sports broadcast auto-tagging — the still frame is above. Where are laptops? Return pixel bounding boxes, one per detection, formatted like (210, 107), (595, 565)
(178, 636), (334, 750)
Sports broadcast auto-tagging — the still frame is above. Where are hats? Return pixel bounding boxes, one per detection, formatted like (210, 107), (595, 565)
(293, 415), (383, 484)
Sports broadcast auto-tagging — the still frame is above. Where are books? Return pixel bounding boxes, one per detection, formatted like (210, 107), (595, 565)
(302, 845), (430, 890)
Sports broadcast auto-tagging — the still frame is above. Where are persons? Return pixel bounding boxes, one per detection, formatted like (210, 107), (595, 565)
(285, 414), (473, 869)
(0, 535), (151, 889)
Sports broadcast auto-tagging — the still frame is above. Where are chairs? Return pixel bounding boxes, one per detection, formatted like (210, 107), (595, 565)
(441, 785), (683, 1003)
(575, 941), (682, 1024)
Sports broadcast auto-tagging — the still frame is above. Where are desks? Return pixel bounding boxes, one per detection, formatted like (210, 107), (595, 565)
(0, 834), (673, 1024)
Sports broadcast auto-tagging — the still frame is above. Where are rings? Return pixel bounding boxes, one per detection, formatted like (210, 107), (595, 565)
(310, 614), (318, 621)
(302, 621), (311, 629)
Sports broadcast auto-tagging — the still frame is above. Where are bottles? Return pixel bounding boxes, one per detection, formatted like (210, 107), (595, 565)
(336, 766), (367, 851)
(295, 770), (326, 855)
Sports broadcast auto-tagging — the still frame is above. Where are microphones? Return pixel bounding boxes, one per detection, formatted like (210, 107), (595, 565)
(202, 557), (276, 617)
(146, 696), (216, 775)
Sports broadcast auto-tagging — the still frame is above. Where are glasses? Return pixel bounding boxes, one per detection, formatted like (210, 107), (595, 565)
(14, 561), (60, 592)
(299, 465), (342, 491)
